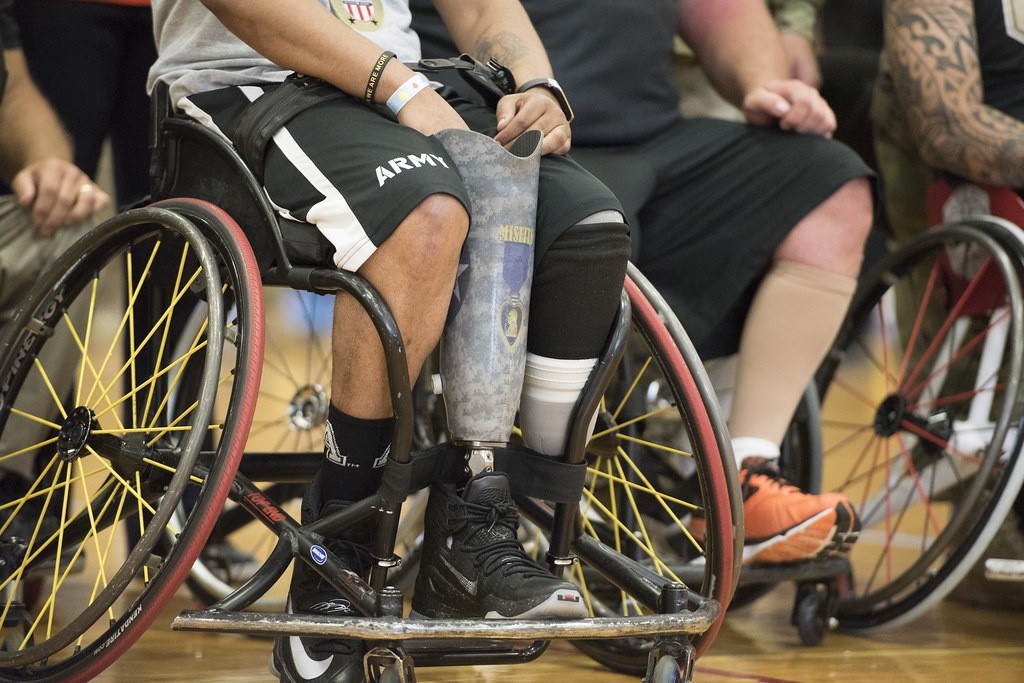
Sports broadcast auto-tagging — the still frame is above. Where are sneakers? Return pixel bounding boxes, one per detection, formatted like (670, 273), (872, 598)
(407, 469), (589, 621)
(268, 466), (372, 683)
(689, 454), (862, 583)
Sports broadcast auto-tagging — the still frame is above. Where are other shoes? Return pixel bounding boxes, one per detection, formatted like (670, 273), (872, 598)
(124, 539), (261, 600)
(0, 506), (84, 578)
(634, 478), (691, 534)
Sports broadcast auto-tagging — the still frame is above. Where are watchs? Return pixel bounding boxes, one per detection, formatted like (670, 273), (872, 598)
(517, 79), (575, 124)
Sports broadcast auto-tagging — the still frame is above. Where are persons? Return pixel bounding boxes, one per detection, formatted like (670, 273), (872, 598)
(0, 0), (1024, 683)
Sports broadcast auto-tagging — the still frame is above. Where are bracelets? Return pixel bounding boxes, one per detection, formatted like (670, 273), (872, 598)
(385, 72), (431, 115)
(364, 51), (397, 105)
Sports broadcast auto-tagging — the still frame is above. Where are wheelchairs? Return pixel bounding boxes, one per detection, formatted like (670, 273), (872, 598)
(0, 52), (1024, 683)
